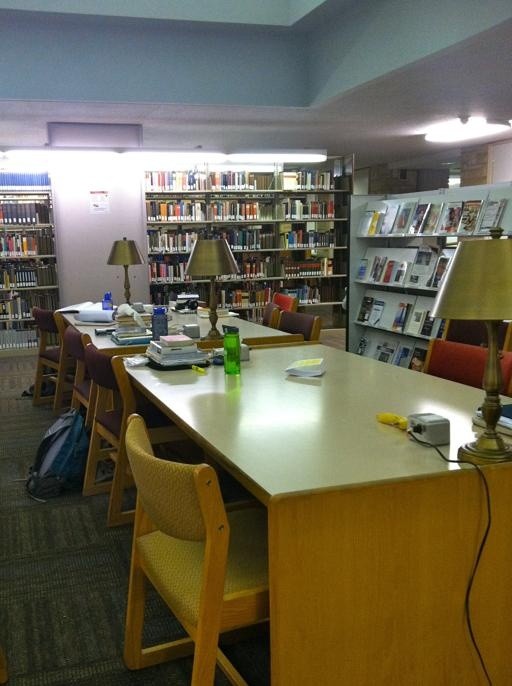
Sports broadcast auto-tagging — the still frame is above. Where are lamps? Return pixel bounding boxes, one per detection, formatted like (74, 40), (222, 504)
(106, 234), (142, 303)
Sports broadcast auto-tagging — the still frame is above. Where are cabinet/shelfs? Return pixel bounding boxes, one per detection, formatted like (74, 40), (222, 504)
(1, 175), (61, 349)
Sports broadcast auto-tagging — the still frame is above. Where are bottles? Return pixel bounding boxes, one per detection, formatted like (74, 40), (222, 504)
(221, 324), (241, 375)
(151, 307), (169, 341)
(101, 291), (114, 310)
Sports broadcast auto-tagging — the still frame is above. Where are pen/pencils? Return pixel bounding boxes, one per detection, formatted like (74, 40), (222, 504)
(191, 365), (205, 373)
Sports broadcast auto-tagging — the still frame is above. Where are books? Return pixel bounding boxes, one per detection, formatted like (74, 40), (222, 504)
(111, 303), (152, 345)
(0, 171), (61, 350)
(357, 195), (508, 371)
(145, 332), (210, 369)
(146, 170), (349, 325)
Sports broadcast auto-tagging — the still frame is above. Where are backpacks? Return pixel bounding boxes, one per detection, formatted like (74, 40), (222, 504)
(25, 408), (90, 501)
